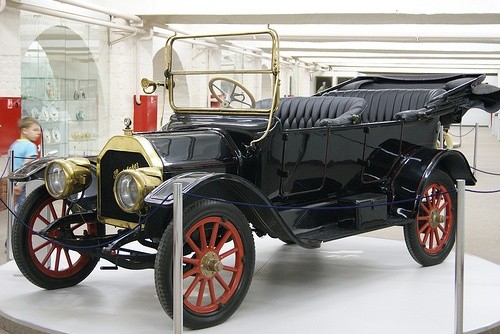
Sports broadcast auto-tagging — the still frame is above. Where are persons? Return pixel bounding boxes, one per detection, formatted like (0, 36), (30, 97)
(3, 117), (48, 262)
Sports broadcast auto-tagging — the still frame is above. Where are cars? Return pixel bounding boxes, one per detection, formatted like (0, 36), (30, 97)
(4, 22), (500, 330)
